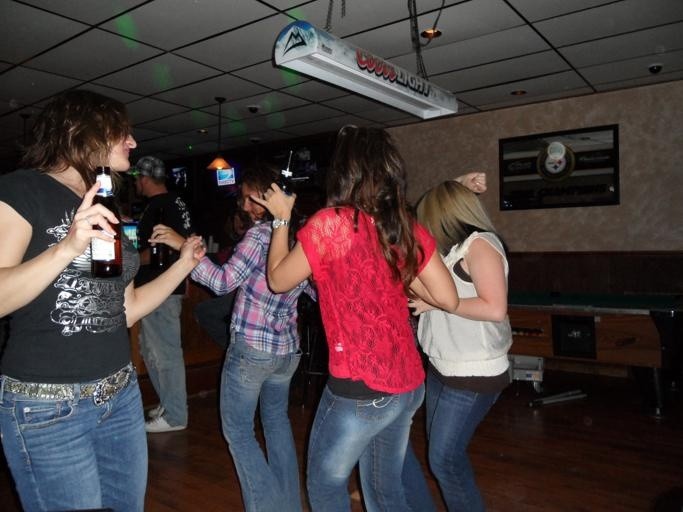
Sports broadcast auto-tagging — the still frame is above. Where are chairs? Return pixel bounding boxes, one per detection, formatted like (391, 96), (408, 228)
(650, 308), (680, 421)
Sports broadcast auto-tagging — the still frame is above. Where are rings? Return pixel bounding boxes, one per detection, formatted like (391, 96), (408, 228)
(85, 215), (93, 226)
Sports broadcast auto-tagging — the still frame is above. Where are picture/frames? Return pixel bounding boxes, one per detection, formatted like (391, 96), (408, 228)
(499, 124), (620, 211)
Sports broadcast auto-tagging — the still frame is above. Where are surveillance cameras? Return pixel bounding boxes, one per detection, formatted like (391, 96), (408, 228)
(247, 105), (260, 113)
(648, 63), (664, 73)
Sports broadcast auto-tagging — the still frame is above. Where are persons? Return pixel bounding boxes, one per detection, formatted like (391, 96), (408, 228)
(408, 171), (514, 512)
(147, 169), (318, 512)
(125, 155), (193, 433)
(0, 90), (207, 512)
(192, 209), (253, 353)
(250, 126), (460, 512)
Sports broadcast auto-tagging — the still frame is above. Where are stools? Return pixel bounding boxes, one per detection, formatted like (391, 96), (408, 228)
(300, 322), (329, 410)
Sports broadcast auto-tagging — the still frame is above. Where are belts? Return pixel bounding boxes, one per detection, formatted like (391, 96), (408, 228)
(5, 361), (135, 408)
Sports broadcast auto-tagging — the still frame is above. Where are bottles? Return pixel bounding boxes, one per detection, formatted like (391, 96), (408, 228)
(88, 166), (124, 279)
(265, 147), (294, 223)
(148, 207), (172, 271)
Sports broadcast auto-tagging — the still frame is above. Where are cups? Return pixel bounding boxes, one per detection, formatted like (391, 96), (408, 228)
(208, 235), (219, 253)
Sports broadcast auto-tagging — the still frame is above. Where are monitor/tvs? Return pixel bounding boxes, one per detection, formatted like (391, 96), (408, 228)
(172, 167), (190, 190)
(216, 167), (237, 186)
(123, 222), (139, 251)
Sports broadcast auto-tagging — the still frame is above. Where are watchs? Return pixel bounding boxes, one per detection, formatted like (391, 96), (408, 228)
(271, 218), (291, 230)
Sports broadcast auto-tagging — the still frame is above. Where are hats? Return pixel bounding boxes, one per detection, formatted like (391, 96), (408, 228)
(125, 155), (166, 177)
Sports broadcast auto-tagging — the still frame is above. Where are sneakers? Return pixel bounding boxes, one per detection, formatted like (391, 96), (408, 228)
(146, 402), (187, 433)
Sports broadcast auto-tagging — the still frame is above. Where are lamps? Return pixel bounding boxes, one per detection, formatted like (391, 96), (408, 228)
(270, 1), (457, 121)
(206, 97), (231, 170)
